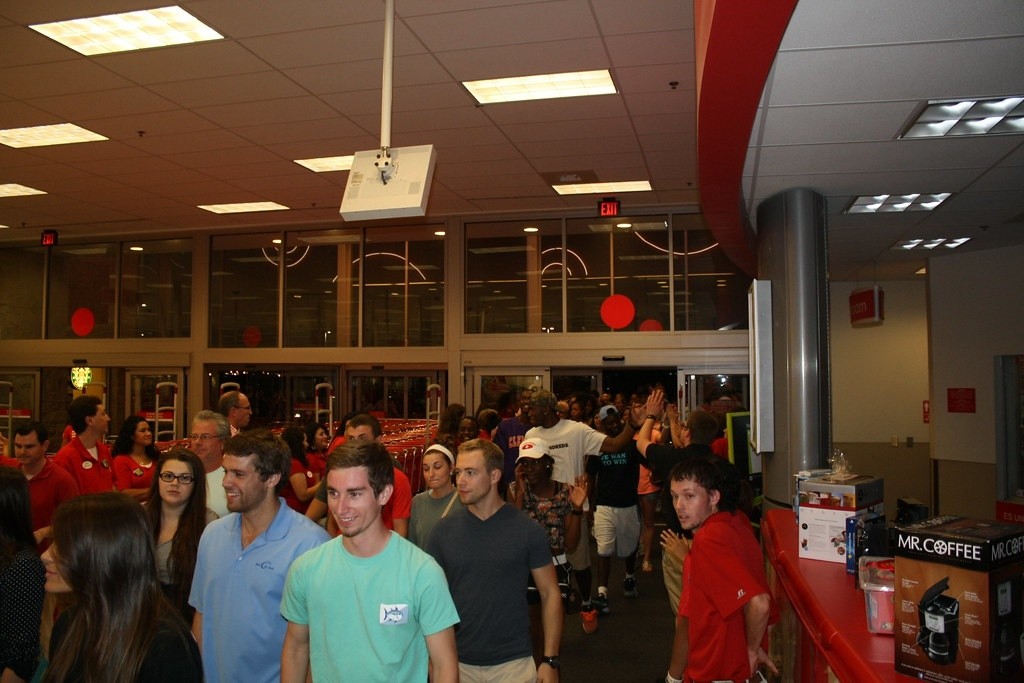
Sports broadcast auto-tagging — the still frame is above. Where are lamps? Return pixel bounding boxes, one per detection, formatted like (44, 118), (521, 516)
(69, 359), (92, 391)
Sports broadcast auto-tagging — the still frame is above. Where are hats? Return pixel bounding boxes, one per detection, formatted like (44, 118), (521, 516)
(515, 437), (554, 465)
(531, 389), (565, 413)
(599, 405), (620, 422)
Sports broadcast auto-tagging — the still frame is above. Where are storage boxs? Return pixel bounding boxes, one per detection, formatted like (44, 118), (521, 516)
(793, 469), (1024, 683)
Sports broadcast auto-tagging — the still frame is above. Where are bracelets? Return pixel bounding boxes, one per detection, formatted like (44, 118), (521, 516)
(572, 510), (583, 515)
(645, 414), (656, 421)
(664, 670), (684, 683)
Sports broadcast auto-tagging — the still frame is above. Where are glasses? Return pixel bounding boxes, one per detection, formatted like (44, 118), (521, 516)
(159, 471), (195, 484)
(191, 434), (219, 440)
(681, 423), (688, 429)
(238, 406), (251, 410)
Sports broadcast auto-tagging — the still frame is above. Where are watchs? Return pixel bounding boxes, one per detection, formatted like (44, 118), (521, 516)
(542, 655), (561, 668)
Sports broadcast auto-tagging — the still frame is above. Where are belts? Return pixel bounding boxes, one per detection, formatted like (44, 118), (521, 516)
(744, 668), (766, 683)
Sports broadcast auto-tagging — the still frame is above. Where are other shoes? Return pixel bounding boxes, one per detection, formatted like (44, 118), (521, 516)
(599, 593), (611, 616)
(623, 578), (636, 596)
(642, 560), (652, 572)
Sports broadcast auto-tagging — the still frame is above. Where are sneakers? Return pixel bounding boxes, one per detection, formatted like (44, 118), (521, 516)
(581, 604), (599, 634)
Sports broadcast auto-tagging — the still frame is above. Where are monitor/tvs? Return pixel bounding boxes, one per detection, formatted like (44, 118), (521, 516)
(339, 145), (436, 222)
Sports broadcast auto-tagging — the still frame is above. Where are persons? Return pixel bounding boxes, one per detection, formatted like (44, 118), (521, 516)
(475, 388), (745, 636)
(457, 416), (476, 442)
(0, 390), (466, 683)
(427, 439), (564, 683)
(664, 457), (779, 683)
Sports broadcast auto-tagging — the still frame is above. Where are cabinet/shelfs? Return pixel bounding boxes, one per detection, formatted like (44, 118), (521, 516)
(760, 509), (926, 683)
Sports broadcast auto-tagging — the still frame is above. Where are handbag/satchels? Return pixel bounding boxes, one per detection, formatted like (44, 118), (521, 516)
(568, 569), (585, 595)
